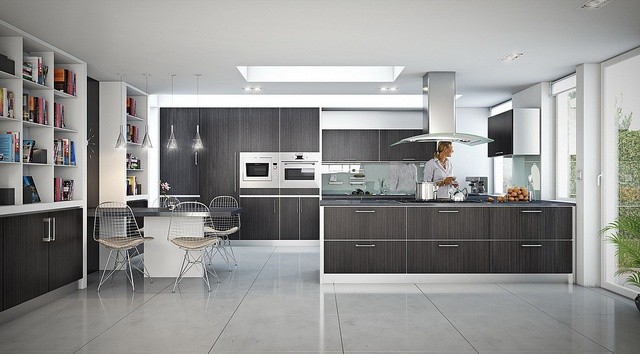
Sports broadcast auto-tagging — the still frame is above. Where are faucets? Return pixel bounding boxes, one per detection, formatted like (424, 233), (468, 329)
(381, 178), (387, 194)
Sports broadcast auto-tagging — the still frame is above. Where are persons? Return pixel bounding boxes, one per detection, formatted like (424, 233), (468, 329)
(424, 141), (459, 199)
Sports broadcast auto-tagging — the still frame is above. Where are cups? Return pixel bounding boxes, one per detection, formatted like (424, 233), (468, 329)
(528, 191), (533, 202)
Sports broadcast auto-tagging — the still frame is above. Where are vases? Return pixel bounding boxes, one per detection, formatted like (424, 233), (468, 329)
(161, 189), (166, 195)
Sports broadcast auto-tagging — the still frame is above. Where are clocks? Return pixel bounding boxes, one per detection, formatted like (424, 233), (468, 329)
(87, 125), (96, 160)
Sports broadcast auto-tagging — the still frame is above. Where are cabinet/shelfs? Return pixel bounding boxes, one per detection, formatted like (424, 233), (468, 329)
(0, 209), (83, 312)
(322, 130), (380, 162)
(407, 207), (489, 284)
(350, 168), (365, 185)
(380, 130), (436, 162)
(0, 20), (87, 215)
(280, 188), (320, 240)
(99, 82), (149, 201)
(240, 108), (280, 151)
(160, 108), (199, 212)
(490, 208), (573, 283)
(126, 199), (148, 237)
(324, 206), (406, 284)
(240, 188), (279, 240)
(280, 108), (319, 152)
(200, 108), (240, 240)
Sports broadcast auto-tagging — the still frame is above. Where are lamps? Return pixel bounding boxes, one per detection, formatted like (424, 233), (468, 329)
(115, 74), (128, 149)
(167, 73), (178, 149)
(192, 73), (204, 149)
(140, 73), (153, 148)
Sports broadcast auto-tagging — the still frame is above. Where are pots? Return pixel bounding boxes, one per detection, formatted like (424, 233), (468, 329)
(415, 179), (439, 201)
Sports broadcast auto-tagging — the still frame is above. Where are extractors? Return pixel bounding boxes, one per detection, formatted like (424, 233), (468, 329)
(389, 71), (494, 147)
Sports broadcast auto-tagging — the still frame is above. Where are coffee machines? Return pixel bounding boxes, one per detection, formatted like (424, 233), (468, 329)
(466, 175), (489, 195)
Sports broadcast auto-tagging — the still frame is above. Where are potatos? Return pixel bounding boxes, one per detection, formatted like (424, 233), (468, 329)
(486, 198), (495, 202)
(502, 196), (507, 201)
(497, 196), (504, 202)
(506, 186), (529, 202)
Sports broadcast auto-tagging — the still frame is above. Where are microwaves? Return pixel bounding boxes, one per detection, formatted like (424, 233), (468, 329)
(239, 151), (279, 188)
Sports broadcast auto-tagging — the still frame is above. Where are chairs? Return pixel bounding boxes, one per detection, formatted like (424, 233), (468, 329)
(93, 201), (154, 292)
(204, 196), (241, 271)
(136, 197), (181, 234)
(167, 202), (222, 292)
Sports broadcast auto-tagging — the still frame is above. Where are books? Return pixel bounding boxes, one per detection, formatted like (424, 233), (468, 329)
(55, 138), (76, 165)
(55, 177), (75, 200)
(1, 131), (20, 161)
(127, 97), (137, 117)
(127, 176), (141, 195)
(0, 88), (14, 117)
(55, 102), (65, 127)
(23, 92), (47, 124)
(24, 176), (40, 203)
(54, 68), (77, 95)
(127, 124), (140, 143)
(22, 55), (45, 84)
(24, 139), (36, 163)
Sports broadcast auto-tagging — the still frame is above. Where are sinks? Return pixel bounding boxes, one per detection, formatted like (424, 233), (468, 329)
(371, 192), (411, 196)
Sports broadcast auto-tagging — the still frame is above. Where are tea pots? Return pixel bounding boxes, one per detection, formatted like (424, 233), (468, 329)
(448, 187), (468, 202)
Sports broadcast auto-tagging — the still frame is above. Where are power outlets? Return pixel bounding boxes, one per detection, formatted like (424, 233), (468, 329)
(576, 171), (581, 181)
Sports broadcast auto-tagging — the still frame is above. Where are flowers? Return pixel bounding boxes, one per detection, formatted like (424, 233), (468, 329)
(161, 182), (171, 191)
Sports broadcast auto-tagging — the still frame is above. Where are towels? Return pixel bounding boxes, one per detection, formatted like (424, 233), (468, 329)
(330, 174), (337, 182)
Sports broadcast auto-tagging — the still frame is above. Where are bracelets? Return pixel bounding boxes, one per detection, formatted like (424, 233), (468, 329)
(443, 178), (447, 186)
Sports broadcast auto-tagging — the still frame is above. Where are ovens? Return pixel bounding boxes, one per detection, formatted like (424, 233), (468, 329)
(279, 152), (321, 188)
